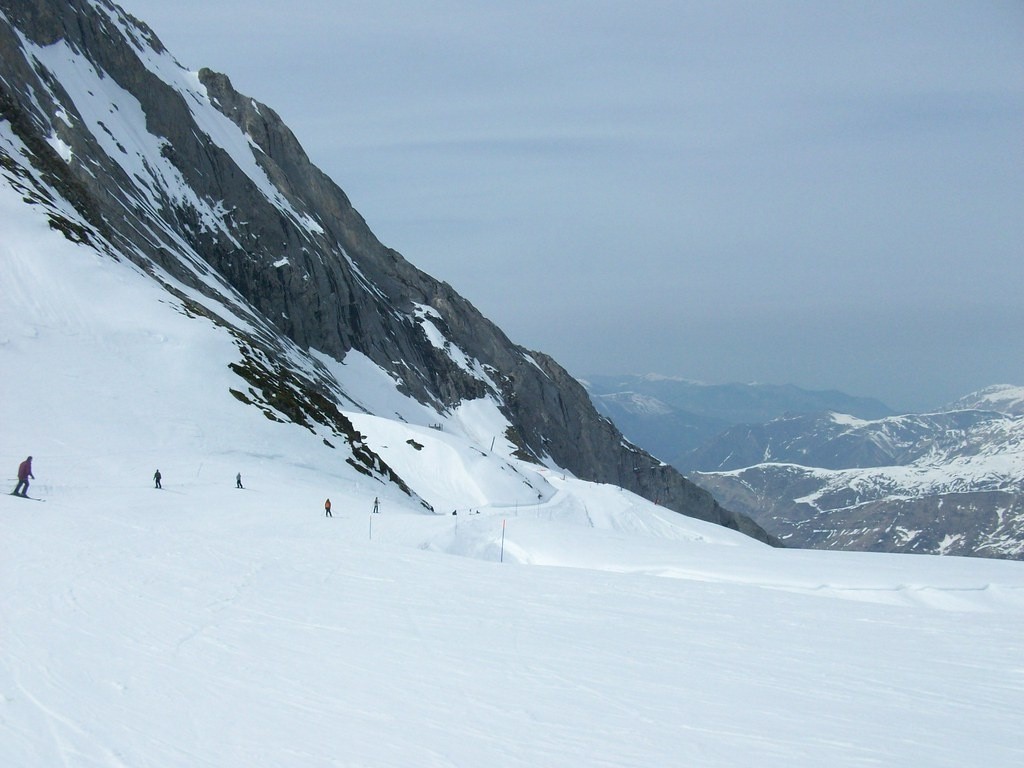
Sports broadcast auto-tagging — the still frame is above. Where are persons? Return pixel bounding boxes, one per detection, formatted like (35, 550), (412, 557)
(13, 456), (34, 498)
(153, 469), (161, 489)
(325, 499), (332, 517)
(236, 473), (243, 488)
(373, 497), (380, 513)
(452, 510), (457, 515)
